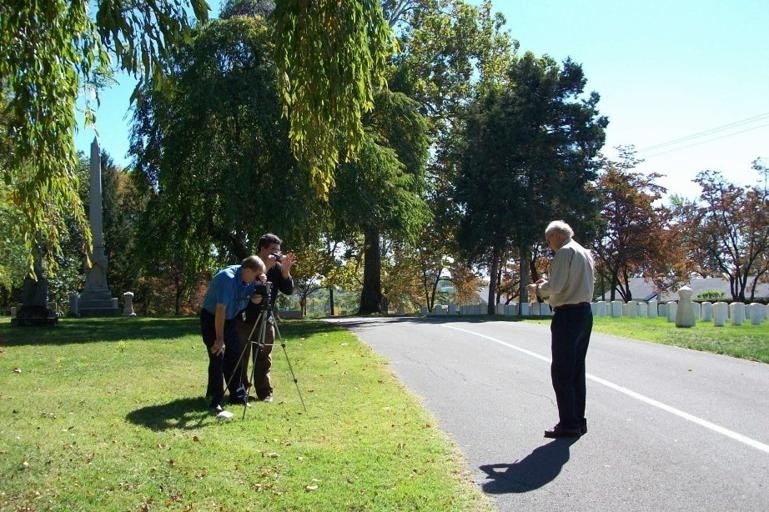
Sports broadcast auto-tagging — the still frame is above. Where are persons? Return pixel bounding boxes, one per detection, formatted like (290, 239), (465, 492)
(526, 219), (594, 438)
(201, 254), (267, 418)
(236, 233), (297, 402)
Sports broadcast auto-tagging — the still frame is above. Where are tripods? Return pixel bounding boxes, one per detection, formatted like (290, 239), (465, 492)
(224, 304), (307, 420)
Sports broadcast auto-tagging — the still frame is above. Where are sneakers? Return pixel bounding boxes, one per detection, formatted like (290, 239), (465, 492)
(260, 390), (272, 401)
(206, 397), (223, 412)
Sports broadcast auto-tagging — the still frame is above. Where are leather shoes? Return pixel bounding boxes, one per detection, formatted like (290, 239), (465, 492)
(545, 423), (587, 437)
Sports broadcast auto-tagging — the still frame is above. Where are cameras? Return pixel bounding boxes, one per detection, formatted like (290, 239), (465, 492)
(272, 254), (280, 261)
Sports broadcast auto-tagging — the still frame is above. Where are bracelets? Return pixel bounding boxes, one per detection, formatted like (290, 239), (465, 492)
(537, 284), (539, 292)
